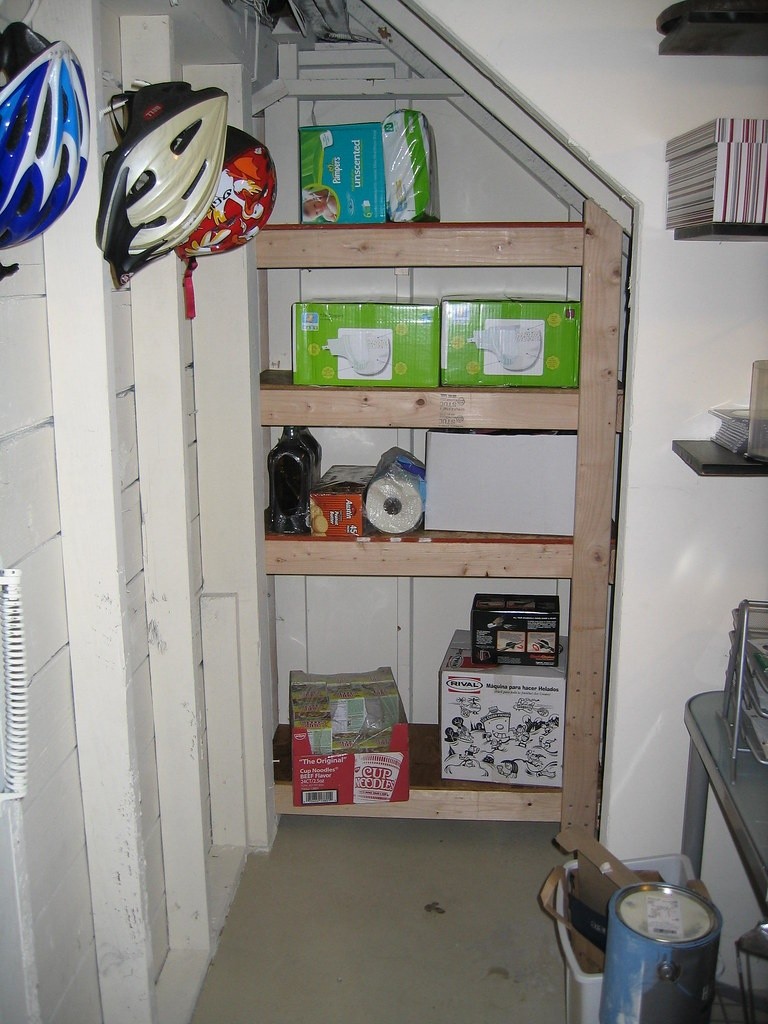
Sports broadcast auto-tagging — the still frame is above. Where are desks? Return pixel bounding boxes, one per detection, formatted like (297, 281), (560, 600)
(682, 690), (768, 918)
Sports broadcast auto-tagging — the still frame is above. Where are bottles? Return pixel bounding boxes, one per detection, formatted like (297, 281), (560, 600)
(267, 426), (323, 534)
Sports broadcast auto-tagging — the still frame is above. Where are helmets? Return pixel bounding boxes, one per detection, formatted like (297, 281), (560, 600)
(95, 81), (229, 289)
(0, 39), (90, 250)
(173, 125), (277, 260)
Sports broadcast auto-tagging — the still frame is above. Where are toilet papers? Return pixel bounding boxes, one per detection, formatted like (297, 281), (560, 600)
(366, 456), (422, 533)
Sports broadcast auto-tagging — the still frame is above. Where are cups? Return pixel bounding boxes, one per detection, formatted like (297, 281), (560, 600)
(746, 360), (768, 463)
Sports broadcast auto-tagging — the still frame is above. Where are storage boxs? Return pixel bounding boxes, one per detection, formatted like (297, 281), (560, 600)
(441, 292), (581, 386)
(291, 294), (441, 385)
(437, 630), (568, 786)
(664, 117), (768, 230)
(423, 428), (578, 536)
(287, 668), (410, 807)
(302, 123), (387, 224)
(470, 593), (560, 668)
(553, 851), (724, 1024)
(309, 464), (377, 537)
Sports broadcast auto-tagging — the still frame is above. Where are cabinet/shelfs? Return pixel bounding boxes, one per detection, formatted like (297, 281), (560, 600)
(722, 599), (768, 787)
(658, 19), (768, 479)
(255, 198), (626, 848)
